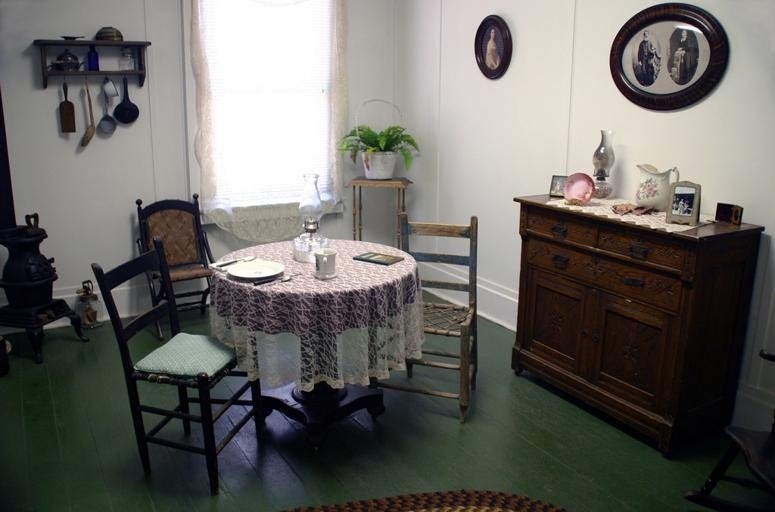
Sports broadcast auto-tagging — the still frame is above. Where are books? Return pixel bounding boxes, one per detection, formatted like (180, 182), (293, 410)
(353, 252), (404, 265)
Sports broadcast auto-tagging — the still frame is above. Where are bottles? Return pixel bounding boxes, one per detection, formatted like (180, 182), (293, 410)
(87, 46), (99, 71)
(119, 47), (135, 70)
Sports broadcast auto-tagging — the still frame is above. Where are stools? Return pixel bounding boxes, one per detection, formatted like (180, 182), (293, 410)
(684, 422), (773, 510)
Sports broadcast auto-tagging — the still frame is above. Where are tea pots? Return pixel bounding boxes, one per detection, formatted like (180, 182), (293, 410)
(47, 48), (85, 71)
(636, 162), (679, 212)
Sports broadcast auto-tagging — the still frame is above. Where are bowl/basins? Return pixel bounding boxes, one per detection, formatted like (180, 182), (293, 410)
(96, 27), (123, 41)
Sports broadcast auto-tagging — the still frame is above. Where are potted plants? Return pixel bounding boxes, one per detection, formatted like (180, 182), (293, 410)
(336, 98), (419, 180)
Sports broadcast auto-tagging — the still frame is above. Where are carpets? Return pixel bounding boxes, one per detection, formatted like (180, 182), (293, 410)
(285, 489), (575, 512)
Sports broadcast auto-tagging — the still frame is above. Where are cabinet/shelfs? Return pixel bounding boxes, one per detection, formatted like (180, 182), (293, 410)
(510, 195), (766, 459)
(22, 38), (152, 88)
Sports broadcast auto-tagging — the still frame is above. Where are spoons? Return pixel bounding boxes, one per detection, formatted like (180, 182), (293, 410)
(217, 255), (257, 267)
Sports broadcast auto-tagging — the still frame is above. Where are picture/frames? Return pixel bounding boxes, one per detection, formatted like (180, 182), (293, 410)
(666, 180), (701, 228)
(474, 14), (512, 81)
(610, 3), (730, 112)
(550, 176), (568, 198)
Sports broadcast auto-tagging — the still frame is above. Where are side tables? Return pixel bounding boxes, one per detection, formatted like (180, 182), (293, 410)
(349, 177), (413, 251)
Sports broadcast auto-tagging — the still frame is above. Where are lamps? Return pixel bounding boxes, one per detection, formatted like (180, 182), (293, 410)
(593, 129), (615, 197)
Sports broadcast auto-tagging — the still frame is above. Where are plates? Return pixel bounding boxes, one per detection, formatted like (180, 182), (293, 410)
(227, 261), (285, 278)
(562, 173), (594, 204)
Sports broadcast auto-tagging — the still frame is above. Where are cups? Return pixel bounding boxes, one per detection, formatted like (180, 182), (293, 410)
(103, 77), (119, 97)
(314, 248), (337, 279)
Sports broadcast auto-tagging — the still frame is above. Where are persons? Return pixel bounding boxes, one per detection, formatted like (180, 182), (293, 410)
(677, 198), (690, 216)
(671, 29), (690, 85)
(638, 30), (660, 85)
(486, 28), (501, 69)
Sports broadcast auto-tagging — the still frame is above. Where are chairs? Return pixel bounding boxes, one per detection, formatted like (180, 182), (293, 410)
(92, 236), (266, 494)
(367, 213), (477, 418)
(135, 195), (217, 339)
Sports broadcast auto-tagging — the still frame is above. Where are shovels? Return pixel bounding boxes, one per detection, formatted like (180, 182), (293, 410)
(79, 80), (96, 147)
(59, 83), (77, 133)
